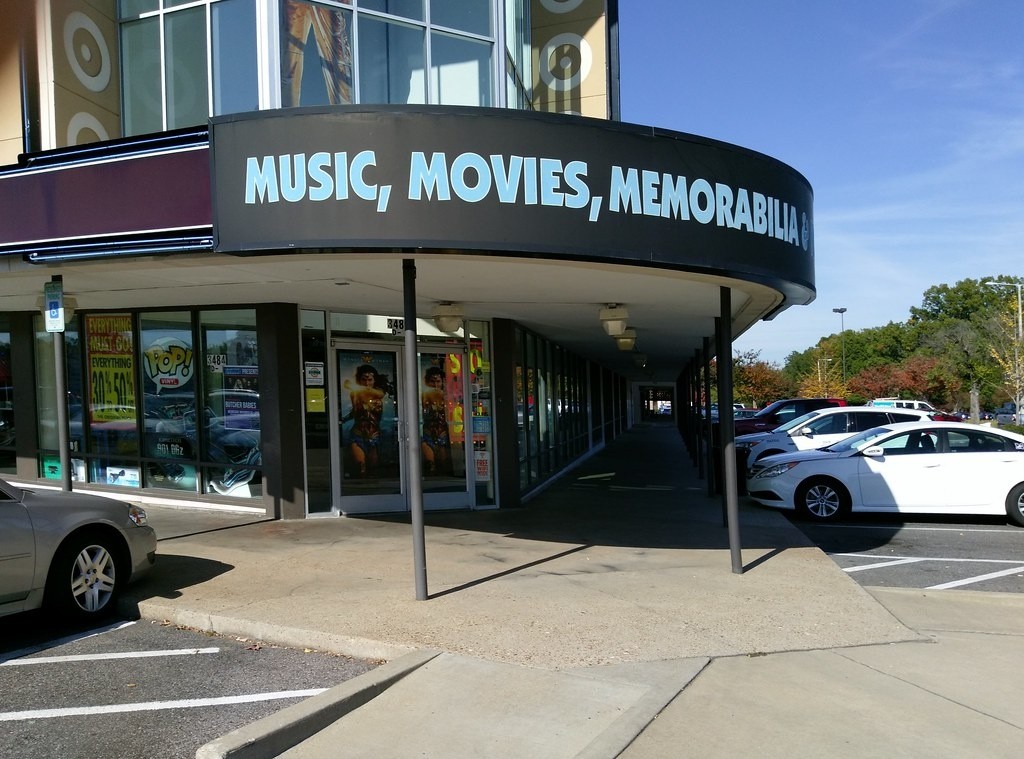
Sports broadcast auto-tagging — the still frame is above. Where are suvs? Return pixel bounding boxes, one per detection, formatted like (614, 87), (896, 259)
(732, 406), (937, 482)
(731, 397), (845, 436)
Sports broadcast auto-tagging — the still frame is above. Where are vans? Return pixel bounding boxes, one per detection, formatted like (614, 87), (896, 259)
(868, 397), (938, 412)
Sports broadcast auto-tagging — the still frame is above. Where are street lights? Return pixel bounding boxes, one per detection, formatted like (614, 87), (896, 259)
(984, 280), (1023, 341)
(817, 357), (831, 391)
(832, 307), (849, 395)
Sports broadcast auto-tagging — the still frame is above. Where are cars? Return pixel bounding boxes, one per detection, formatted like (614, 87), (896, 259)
(659, 394), (760, 425)
(747, 422), (1024, 527)
(0, 473), (157, 647)
(919, 406), (1017, 423)
(0, 384), (265, 501)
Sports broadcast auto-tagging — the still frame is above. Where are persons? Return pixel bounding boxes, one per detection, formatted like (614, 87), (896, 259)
(339, 366), (386, 474)
(421, 366), (448, 476)
